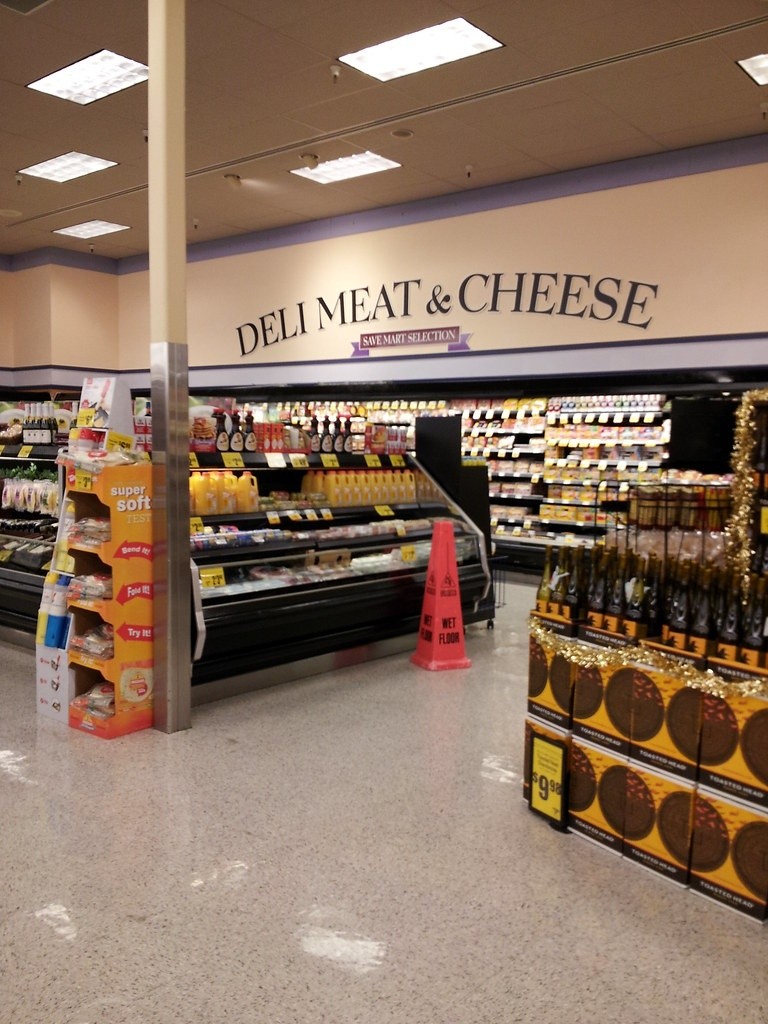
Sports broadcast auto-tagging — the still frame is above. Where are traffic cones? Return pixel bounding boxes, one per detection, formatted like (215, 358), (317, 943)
(408, 519), (471, 670)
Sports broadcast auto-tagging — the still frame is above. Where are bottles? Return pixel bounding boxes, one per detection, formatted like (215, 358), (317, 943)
(301, 468), (417, 508)
(212, 408), (258, 452)
(189, 470), (260, 516)
(541, 540), (768, 671)
(309, 414), (353, 453)
(22, 403), (58, 446)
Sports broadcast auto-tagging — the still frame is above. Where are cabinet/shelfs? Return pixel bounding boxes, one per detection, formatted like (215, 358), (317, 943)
(0, 384), (674, 739)
